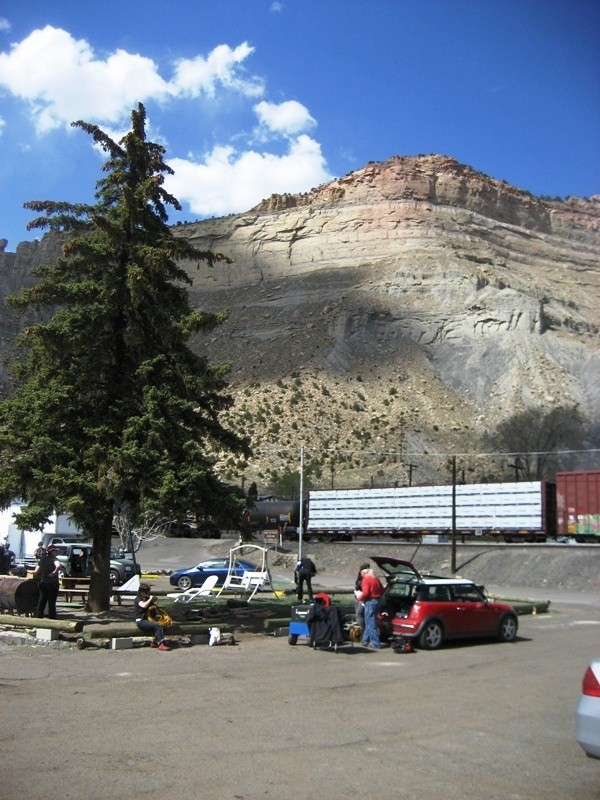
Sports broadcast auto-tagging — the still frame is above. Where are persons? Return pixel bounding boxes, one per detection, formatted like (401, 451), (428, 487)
(0, 541), (61, 619)
(135, 583), (170, 650)
(294, 558), (318, 603)
(355, 567), (385, 648)
(354, 561), (371, 642)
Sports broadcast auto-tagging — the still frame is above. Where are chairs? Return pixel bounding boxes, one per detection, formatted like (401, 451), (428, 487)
(166, 575), (219, 603)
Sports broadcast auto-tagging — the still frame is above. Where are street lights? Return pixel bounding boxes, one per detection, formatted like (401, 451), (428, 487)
(447, 454), (469, 574)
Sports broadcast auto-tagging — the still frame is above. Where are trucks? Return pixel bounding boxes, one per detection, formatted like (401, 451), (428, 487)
(0, 505), (88, 577)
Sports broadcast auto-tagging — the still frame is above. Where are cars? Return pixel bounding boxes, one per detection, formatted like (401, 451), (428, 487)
(368, 555), (520, 652)
(169, 557), (264, 591)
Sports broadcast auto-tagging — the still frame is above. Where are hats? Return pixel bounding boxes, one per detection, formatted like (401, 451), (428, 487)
(360, 563), (370, 570)
(47, 545), (60, 552)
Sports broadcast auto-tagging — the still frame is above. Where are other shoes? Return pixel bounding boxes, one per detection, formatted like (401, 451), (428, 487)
(159, 642), (171, 650)
(368, 642), (380, 648)
(151, 642), (158, 648)
(360, 641), (369, 646)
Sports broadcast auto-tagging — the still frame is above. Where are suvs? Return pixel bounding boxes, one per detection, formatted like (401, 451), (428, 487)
(45, 542), (141, 584)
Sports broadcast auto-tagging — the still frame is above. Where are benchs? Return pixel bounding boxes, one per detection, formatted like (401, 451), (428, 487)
(263, 618), (293, 636)
(59, 589), (88, 603)
(0, 614), (82, 641)
(84, 623), (232, 648)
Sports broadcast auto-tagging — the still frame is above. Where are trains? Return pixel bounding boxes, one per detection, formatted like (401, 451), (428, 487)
(241, 468), (599, 544)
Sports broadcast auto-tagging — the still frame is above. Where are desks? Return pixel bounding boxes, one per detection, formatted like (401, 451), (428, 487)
(58, 577), (90, 602)
